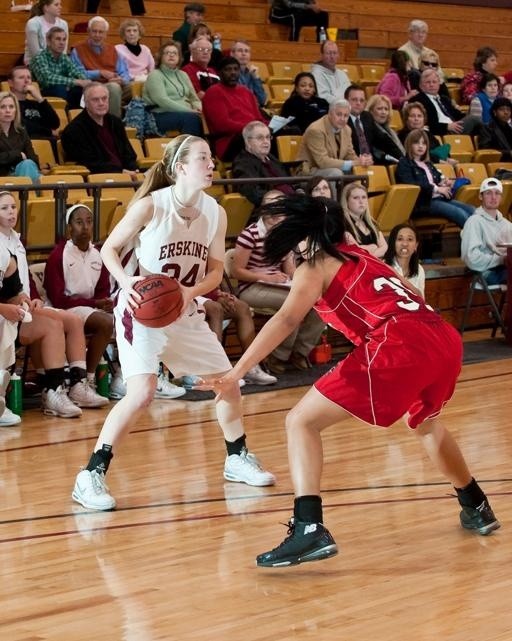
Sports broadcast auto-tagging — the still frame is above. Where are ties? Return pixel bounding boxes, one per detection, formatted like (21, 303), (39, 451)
(433, 98), (453, 121)
(355, 118), (368, 155)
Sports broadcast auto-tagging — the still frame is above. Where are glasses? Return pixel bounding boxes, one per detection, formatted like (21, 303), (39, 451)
(163, 50), (178, 54)
(193, 47), (210, 54)
(251, 133), (274, 142)
(423, 61), (437, 68)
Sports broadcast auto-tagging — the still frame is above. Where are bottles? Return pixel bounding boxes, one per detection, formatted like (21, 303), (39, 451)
(97, 355), (109, 398)
(319, 26), (327, 45)
(213, 35), (221, 50)
(6, 374), (23, 417)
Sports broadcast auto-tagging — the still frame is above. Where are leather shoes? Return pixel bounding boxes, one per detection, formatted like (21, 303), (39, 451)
(288, 351), (308, 371)
(264, 355), (286, 374)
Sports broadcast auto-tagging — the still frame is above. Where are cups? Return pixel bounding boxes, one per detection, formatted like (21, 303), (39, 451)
(327, 28), (338, 41)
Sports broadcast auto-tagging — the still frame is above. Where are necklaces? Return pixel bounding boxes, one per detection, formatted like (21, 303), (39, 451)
(172, 189), (201, 209)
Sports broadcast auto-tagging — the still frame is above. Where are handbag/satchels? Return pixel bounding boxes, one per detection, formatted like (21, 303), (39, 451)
(308, 334), (333, 364)
(449, 168), (471, 196)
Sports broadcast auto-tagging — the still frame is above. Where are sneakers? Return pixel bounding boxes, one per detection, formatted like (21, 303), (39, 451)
(256, 516), (338, 567)
(181, 375), (212, 391)
(223, 447), (276, 486)
(446, 489), (501, 535)
(31, 367), (126, 417)
(153, 373), (186, 399)
(243, 364), (278, 385)
(0, 407), (22, 426)
(71, 465), (117, 511)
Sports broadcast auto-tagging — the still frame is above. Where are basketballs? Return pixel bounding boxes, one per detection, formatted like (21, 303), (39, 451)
(130, 275), (182, 328)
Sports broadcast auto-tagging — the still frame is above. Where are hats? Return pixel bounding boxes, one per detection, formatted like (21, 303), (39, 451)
(479, 177), (503, 194)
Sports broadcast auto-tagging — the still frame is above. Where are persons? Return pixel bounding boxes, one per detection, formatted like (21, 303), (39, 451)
(72, 134), (277, 511)
(1, 0), (512, 426)
(192, 192), (500, 568)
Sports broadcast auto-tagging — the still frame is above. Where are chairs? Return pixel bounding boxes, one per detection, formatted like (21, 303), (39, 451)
(0, 0), (512, 403)
